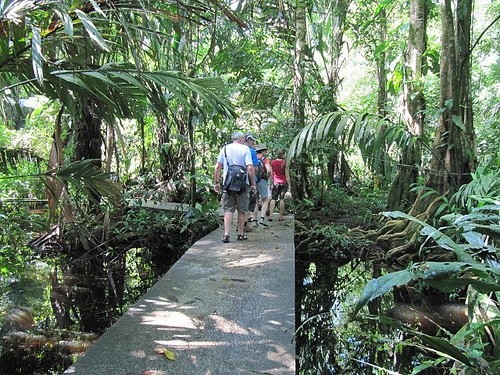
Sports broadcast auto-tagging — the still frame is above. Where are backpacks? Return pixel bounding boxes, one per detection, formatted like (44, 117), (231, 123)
(257, 156), (267, 181)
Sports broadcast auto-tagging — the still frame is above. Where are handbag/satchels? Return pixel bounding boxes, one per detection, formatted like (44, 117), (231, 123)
(223, 164), (247, 194)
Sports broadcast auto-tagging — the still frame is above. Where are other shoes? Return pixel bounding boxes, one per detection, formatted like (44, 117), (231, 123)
(221, 234), (230, 243)
(278, 217), (285, 221)
(235, 222), (252, 232)
(268, 216), (273, 221)
(253, 219), (259, 227)
(259, 216), (269, 226)
(248, 216), (254, 222)
(237, 233), (248, 241)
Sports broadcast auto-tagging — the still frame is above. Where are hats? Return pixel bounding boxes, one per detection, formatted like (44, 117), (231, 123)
(255, 143), (268, 151)
(244, 132), (257, 142)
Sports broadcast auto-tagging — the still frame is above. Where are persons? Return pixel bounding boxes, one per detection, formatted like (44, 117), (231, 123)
(214, 132), (257, 243)
(237, 135), (289, 231)
